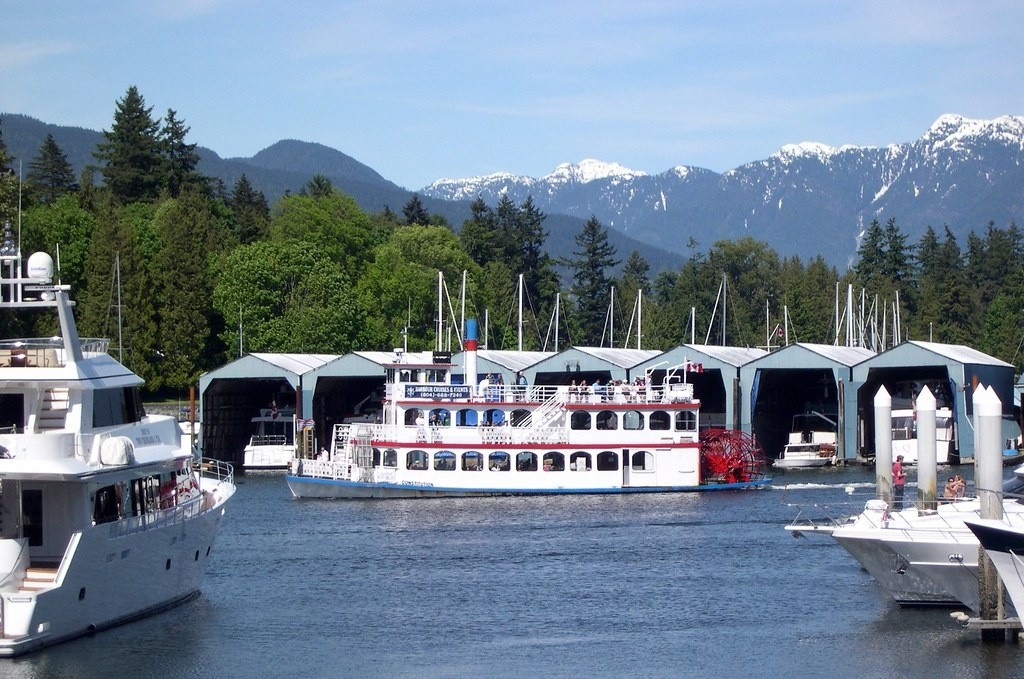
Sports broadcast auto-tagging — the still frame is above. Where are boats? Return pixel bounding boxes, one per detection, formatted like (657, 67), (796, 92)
(240, 393), (298, 473)
(284, 318), (773, 499)
(949, 488), (1024, 630)
(771, 398), (838, 467)
(893, 368), (952, 464)
(0, 161), (236, 657)
(786, 382), (1024, 610)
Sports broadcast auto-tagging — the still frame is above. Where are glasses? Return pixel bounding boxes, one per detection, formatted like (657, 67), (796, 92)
(949, 481), (954, 482)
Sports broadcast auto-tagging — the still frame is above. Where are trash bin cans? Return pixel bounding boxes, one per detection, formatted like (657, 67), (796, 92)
(10, 347), (28, 367)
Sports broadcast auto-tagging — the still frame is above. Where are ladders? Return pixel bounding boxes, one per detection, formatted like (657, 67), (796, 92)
(356, 427), (373, 482)
(303, 427), (315, 461)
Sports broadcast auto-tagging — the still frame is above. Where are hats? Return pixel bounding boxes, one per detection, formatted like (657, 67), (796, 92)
(896, 455), (904, 459)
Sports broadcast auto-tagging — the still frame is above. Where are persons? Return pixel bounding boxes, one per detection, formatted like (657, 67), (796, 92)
(891, 455), (908, 507)
(317, 372), (653, 471)
(938, 474), (967, 504)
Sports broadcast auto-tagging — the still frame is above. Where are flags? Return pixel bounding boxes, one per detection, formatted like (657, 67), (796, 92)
(686, 360), (703, 373)
(776, 327), (784, 338)
